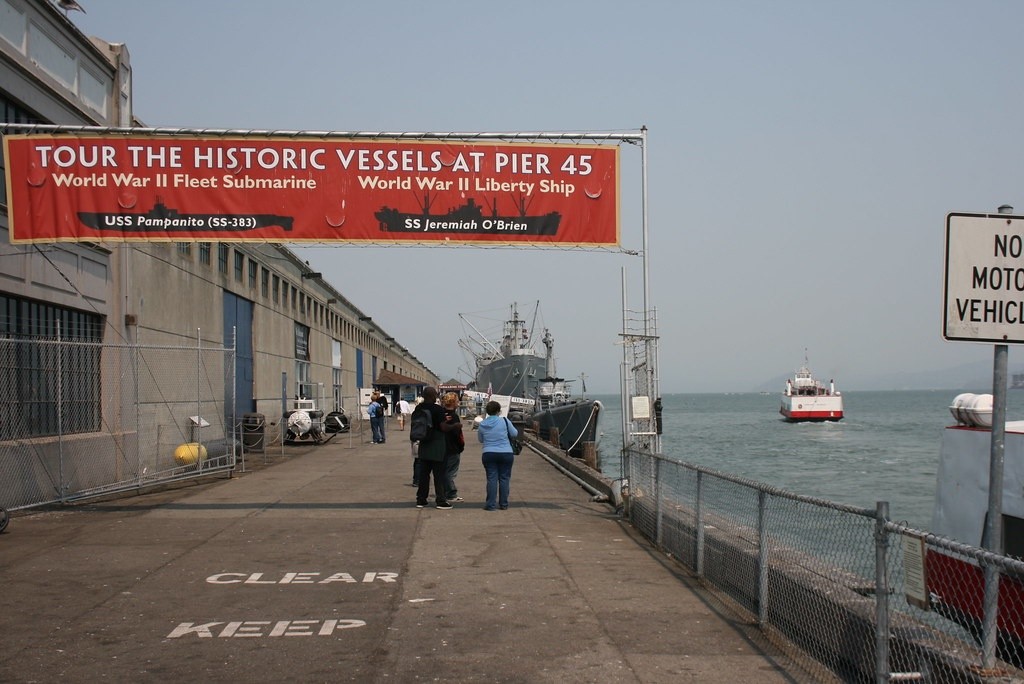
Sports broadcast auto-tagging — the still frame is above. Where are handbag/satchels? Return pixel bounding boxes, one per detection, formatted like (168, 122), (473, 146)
(510, 436), (522, 456)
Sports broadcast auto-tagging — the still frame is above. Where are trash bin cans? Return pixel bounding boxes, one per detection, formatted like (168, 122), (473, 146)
(243, 413), (265, 452)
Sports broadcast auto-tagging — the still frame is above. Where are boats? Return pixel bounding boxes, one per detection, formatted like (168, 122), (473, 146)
(455, 300), (572, 425)
(778, 347), (845, 421)
(526, 328), (602, 456)
(923, 389), (1023, 655)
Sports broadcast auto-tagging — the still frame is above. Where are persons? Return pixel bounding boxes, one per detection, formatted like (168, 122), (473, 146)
(366, 386), (466, 509)
(477, 401), (519, 510)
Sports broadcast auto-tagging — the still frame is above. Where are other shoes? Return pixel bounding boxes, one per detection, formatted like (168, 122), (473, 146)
(447, 497), (464, 503)
(500, 505), (507, 510)
(412, 483), (419, 487)
(416, 502), (430, 508)
(484, 506), (496, 511)
(436, 503), (454, 510)
(370, 441), (377, 445)
(380, 439), (383, 442)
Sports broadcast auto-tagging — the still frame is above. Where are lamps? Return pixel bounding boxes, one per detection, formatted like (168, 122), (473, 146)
(302, 272), (322, 279)
(359, 317), (372, 321)
(385, 338), (394, 341)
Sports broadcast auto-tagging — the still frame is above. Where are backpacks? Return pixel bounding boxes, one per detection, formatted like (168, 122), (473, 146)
(370, 402), (383, 418)
(396, 401), (401, 414)
(409, 402), (441, 443)
